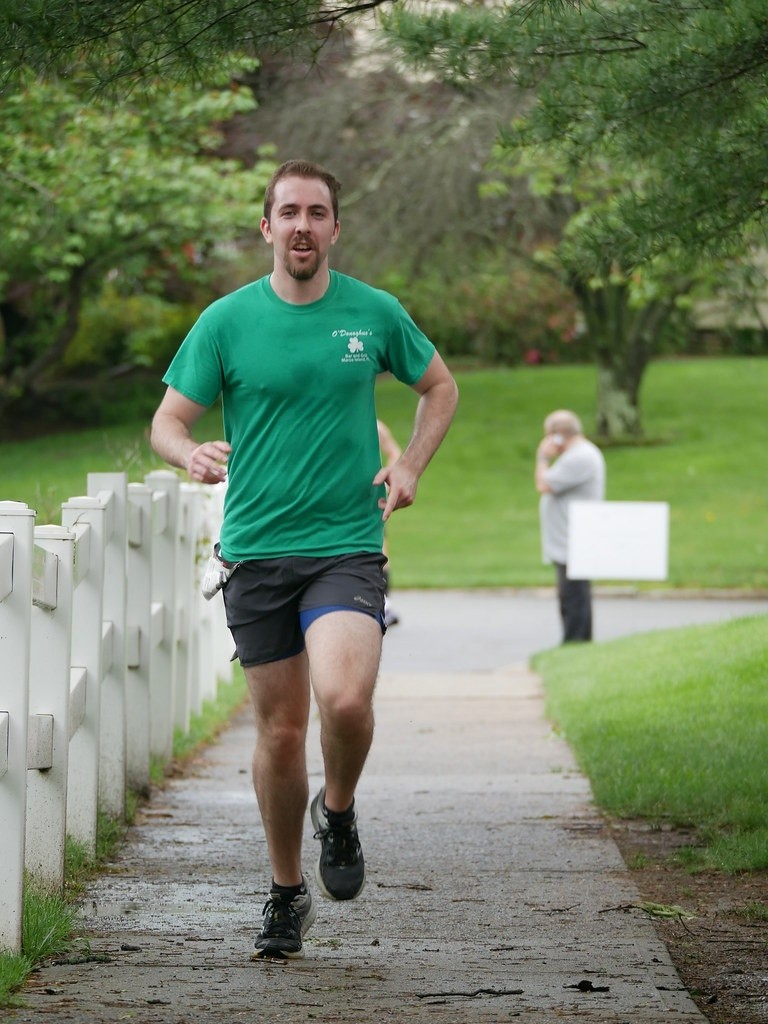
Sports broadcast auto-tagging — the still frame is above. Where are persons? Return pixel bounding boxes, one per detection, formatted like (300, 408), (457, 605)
(533, 409), (604, 648)
(148, 160), (460, 959)
(374, 416), (405, 633)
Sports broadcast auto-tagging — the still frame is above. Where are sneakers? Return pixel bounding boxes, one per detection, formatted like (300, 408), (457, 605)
(309, 784), (367, 901)
(254, 874), (318, 959)
